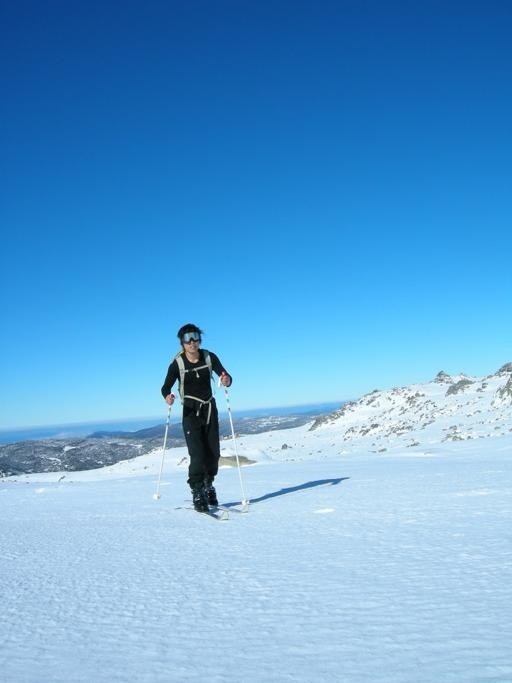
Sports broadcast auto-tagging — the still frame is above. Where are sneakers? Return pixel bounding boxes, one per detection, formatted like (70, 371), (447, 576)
(191, 481), (217, 506)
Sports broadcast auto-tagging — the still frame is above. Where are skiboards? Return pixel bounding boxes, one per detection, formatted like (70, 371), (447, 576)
(174, 500), (250, 521)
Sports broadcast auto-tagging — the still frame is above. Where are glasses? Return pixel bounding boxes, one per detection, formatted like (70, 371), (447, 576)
(181, 330), (201, 343)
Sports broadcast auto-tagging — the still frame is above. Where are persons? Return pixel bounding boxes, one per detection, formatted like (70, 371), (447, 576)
(160, 324), (233, 513)
